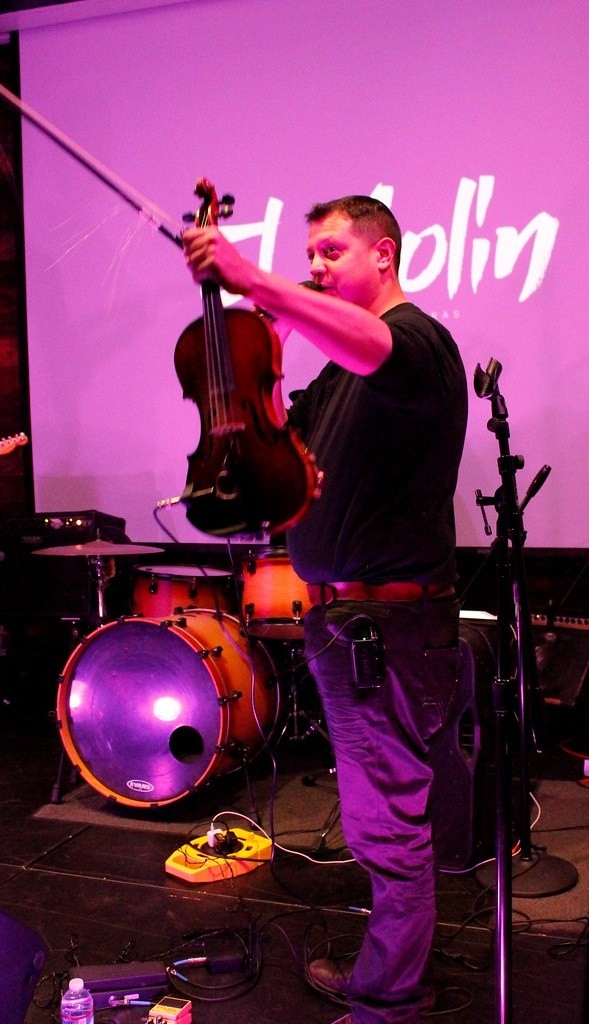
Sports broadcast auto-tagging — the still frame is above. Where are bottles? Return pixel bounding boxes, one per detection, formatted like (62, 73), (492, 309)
(60, 977), (94, 1024)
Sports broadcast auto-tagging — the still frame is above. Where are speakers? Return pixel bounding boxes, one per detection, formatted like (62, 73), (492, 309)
(424, 618), (515, 872)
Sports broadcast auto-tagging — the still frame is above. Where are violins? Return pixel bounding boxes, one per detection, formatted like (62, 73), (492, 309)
(172, 174), (324, 542)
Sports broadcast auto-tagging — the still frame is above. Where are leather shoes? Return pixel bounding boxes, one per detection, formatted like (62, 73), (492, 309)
(308, 958), (436, 1007)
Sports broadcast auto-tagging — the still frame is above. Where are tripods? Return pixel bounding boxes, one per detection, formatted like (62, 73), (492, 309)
(277, 640), (332, 749)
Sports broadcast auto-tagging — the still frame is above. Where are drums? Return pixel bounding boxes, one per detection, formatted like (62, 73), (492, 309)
(130, 563), (234, 618)
(240, 545), (312, 641)
(53, 608), (282, 809)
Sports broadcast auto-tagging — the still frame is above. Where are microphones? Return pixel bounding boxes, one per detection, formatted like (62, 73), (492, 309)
(260, 281), (323, 321)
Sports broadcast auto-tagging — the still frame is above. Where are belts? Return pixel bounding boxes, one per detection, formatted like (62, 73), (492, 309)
(305, 581), (456, 606)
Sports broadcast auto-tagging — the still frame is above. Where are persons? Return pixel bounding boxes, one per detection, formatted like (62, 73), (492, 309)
(178, 195), (469, 1024)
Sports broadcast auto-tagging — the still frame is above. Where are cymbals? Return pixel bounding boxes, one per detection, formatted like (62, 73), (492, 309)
(29, 537), (166, 557)
